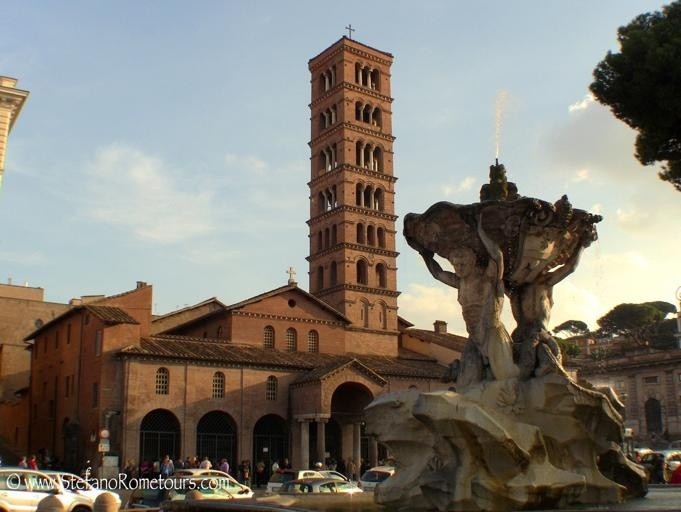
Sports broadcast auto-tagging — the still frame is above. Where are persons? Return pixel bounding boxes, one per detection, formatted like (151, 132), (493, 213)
(511, 227), (587, 381)
(1, 444), (393, 482)
(412, 216), (521, 383)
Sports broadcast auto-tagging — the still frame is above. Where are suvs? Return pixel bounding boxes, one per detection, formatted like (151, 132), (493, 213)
(358, 466), (396, 490)
(1, 469), (93, 512)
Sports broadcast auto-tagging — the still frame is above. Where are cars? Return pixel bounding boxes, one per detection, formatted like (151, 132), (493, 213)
(636, 448), (681, 484)
(124, 482), (235, 511)
(265, 470), (324, 494)
(278, 478), (367, 493)
(42, 471), (123, 512)
(187, 475), (253, 500)
(318, 469), (359, 487)
(171, 469), (238, 484)
